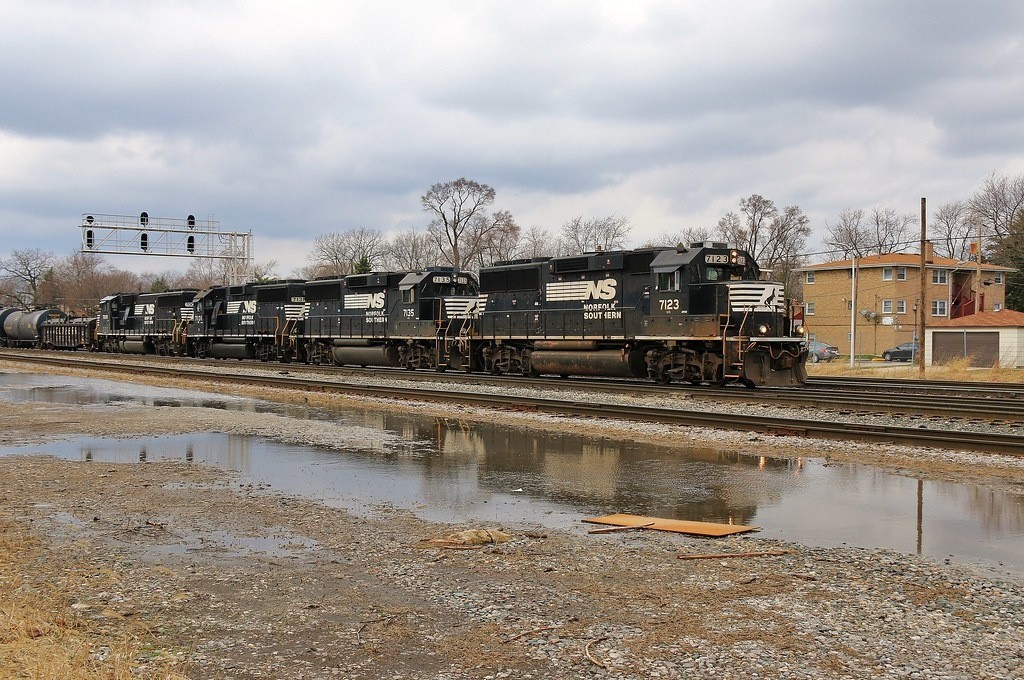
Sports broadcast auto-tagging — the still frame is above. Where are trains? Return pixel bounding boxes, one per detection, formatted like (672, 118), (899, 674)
(0, 237), (813, 388)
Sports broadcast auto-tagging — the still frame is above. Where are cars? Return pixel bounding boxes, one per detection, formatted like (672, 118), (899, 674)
(800, 341), (841, 364)
(881, 341), (920, 362)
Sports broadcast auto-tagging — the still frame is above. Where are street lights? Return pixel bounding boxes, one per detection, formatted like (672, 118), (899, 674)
(829, 241), (856, 367)
